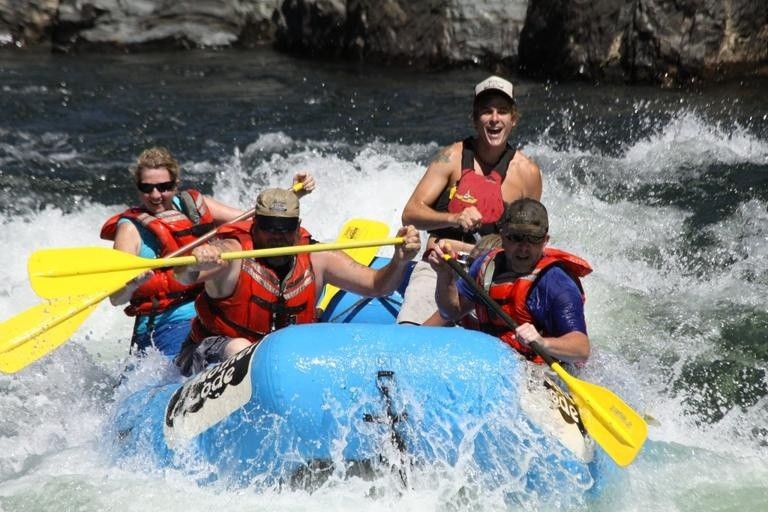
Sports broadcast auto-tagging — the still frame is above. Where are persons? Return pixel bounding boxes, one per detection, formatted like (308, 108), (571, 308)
(394, 75), (543, 326)
(167, 186), (423, 385)
(427, 196), (591, 394)
(106, 144), (317, 360)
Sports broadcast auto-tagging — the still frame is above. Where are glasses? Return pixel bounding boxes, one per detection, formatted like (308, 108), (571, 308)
(504, 233), (544, 244)
(136, 181), (172, 193)
(257, 218), (296, 234)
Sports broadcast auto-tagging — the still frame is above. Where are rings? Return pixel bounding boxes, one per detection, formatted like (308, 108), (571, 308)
(201, 249), (207, 256)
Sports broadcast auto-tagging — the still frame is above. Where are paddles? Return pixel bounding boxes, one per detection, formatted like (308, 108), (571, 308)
(314, 218), (392, 323)
(2, 176), (312, 373)
(441, 241), (649, 467)
(27, 229), (409, 302)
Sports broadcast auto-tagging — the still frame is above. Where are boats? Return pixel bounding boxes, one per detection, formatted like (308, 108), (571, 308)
(99, 251), (649, 508)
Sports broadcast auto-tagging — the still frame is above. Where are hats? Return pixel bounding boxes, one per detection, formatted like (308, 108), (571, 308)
(254, 188), (299, 219)
(473, 75), (514, 103)
(502, 198), (548, 237)
